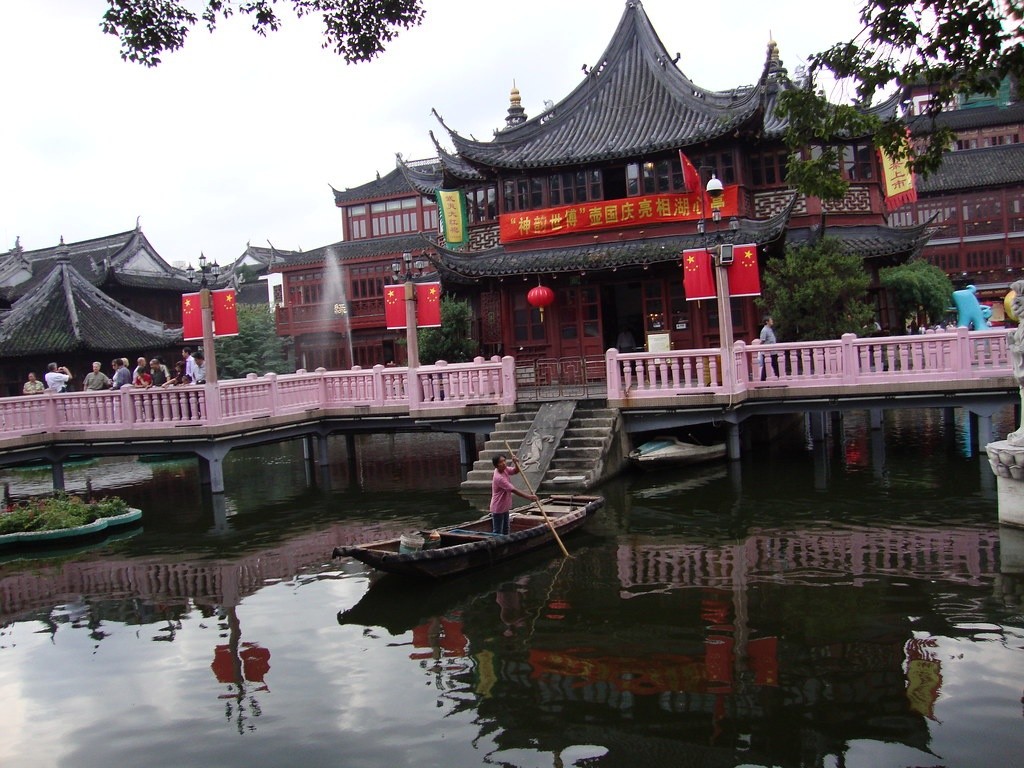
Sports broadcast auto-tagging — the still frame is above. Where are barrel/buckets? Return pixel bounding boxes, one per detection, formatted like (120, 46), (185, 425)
(399, 534), (425, 554)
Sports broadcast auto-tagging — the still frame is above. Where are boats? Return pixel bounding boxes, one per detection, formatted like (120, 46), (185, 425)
(624, 433), (728, 470)
(333, 494), (606, 579)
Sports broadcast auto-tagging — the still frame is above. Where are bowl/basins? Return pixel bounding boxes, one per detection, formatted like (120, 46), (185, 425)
(423, 537), (441, 550)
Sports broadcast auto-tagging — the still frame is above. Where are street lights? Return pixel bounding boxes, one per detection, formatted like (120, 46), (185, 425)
(186, 253), (220, 383)
(696, 207), (740, 348)
(391, 250), (429, 368)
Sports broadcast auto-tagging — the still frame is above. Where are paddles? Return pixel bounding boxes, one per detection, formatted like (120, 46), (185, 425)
(503, 438), (570, 556)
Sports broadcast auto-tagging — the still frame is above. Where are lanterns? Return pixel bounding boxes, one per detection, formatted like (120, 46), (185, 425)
(527, 285), (554, 323)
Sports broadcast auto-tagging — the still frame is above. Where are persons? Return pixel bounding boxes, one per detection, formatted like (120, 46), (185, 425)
(489, 454), (538, 536)
(21, 346), (207, 422)
(918, 318), (993, 334)
(758, 317), (779, 383)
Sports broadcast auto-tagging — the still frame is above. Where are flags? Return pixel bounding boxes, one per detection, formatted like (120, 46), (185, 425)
(182, 293), (204, 341)
(727, 243), (761, 297)
(383, 285), (407, 330)
(681, 249), (717, 301)
(211, 288), (239, 337)
(414, 282), (443, 329)
(678, 150), (701, 195)
